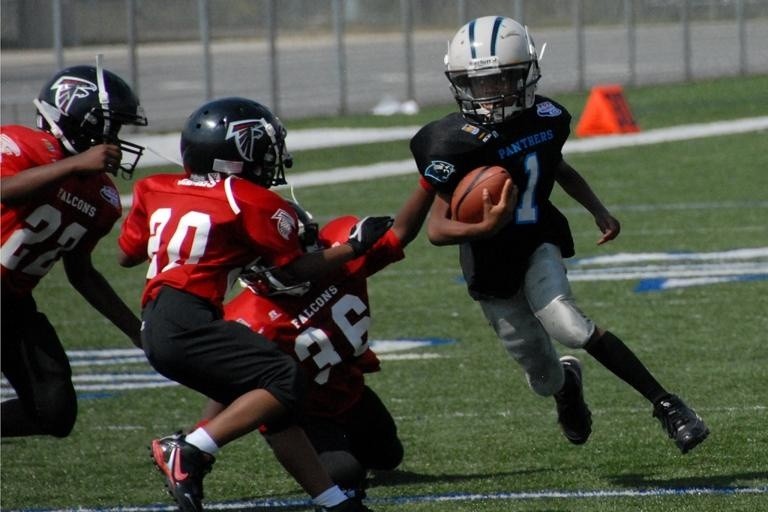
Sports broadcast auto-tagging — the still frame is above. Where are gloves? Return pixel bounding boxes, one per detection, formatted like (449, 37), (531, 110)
(346, 215), (396, 259)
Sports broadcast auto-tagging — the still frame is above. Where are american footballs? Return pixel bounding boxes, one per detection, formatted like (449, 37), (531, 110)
(450, 165), (510, 224)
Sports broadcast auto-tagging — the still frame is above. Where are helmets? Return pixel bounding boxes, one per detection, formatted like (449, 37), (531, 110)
(33, 64), (146, 181)
(237, 200), (319, 299)
(445, 15), (542, 126)
(180, 98), (291, 190)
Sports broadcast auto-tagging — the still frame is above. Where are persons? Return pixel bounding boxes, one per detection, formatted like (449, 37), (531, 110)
(428, 16), (709, 454)
(119, 97), (371, 511)
(0, 64), (145, 439)
(221, 138), (461, 489)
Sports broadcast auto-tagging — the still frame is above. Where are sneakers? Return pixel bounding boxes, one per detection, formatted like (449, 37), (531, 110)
(556, 354), (592, 446)
(148, 432), (215, 512)
(656, 396), (710, 454)
(317, 498), (369, 512)
